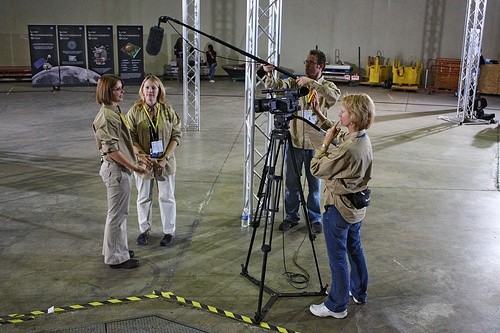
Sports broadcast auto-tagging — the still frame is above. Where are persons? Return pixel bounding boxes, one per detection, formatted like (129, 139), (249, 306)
(92, 73), (153, 269)
(174, 38), (183, 83)
(470, 49), (485, 99)
(206, 44), (218, 82)
(311, 93), (376, 317)
(260, 49), (341, 234)
(187, 47), (203, 68)
(127, 75), (182, 245)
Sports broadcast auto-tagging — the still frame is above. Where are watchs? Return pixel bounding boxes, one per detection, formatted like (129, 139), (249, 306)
(163, 155), (170, 161)
(320, 144), (329, 149)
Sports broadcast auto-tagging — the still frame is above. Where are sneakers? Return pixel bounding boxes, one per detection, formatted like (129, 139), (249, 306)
(349, 290), (363, 304)
(310, 302), (347, 319)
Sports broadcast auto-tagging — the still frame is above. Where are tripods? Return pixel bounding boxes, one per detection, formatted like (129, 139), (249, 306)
(239, 129), (329, 325)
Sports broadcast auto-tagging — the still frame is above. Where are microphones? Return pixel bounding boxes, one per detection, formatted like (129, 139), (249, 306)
(146, 18), (164, 56)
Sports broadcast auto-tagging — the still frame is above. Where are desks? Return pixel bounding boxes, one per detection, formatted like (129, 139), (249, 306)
(323, 66), (353, 86)
(477, 64), (500, 94)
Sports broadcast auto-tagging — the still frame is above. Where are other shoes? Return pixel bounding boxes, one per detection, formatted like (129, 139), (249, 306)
(311, 222), (322, 233)
(279, 220), (299, 231)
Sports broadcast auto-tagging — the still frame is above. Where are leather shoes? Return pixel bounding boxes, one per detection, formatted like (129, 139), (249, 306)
(137, 233), (150, 245)
(128, 250), (134, 257)
(160, 234), (175, 246)
(109, 258), (139, 269)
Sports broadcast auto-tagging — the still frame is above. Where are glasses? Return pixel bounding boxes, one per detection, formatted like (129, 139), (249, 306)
(302, 60), (319, 66)
(112, 86), (124, 91)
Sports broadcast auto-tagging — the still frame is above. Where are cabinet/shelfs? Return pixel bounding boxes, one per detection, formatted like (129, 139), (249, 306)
(434, 59), (460, 89)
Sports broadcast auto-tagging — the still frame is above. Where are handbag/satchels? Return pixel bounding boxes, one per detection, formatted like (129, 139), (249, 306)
(344, 189), (371, 209)
